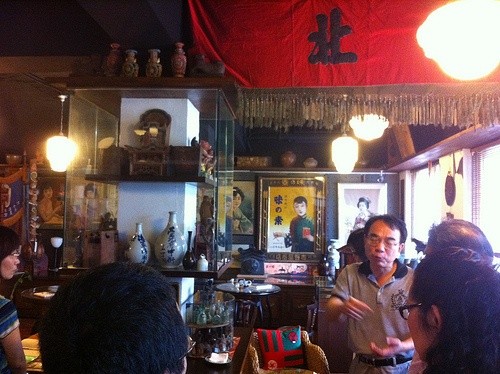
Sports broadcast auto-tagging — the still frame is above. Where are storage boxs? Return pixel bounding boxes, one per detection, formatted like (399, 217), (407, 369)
(237, 157), (272, 168)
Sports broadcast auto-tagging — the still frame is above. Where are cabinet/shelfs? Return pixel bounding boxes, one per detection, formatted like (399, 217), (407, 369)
(188, 321), (233, 359)
(0, 76), (238, 277)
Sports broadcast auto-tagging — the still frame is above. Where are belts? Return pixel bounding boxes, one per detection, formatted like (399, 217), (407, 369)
(355, 353), (412, 367)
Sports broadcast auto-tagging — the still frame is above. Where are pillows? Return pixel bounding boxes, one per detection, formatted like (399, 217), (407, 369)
(256, 325), (309, 370)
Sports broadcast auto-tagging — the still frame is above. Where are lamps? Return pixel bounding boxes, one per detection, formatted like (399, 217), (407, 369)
(331, 94), (389, 173)
(416, 0), (500, 81)
(46, 96), (78, 173)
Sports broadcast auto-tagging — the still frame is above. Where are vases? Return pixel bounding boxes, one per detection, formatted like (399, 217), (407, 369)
(125, 222), (150, 266)
(154, 212), (183, 268)
(183, 231), (196, 270)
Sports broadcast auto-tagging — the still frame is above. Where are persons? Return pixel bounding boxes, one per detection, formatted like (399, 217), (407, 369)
(324, 212), (416, 374)
(36, 259), (192, 374)
(396, 245), (500, 374)
(0, 223), (28, 374)
(406, 217), (495, 373)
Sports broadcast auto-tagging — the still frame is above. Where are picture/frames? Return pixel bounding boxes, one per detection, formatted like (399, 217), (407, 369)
(37, 177), (64, 229)
(325, 172), (399, 257)
(233, 172), (257, 245)
(258, 176), (325, 262)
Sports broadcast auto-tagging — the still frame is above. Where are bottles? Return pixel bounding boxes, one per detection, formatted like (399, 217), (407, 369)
(326, 239), (340, 276)
(303, 157), (318, 168)
(357, 161), (367, 168)
(280, 151), (295, 169)
(197, 254), (208, 272)
(128, 222), (151, 266)
(118, 50), (138, 78)
(193, 326), (233, 354)
(186, 288), (233, 325)
(170, 42), (186, 78)
(145, 49), (162, 78)
(154, 211), (188, 269)
(182, 231), (196, 270)
(103, 43), (121, 76)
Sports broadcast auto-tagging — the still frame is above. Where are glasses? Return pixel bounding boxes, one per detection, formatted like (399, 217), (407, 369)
(9, 248), (21, 260)
(366, 236), (400, 249)
(399, 299), (447, 321)
(177, 335), (196, 360)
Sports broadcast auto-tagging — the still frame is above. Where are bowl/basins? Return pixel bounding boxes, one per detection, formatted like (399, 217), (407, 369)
(6, 155), (22, 164)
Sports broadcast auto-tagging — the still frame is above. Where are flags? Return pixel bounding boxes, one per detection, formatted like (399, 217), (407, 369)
(0, 148), (30, 227)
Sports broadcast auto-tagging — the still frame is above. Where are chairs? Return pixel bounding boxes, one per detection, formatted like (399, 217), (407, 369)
(240, 331), (329, 374)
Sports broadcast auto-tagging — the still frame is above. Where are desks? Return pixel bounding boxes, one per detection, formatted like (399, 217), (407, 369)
(215, 283), (281, 327)
